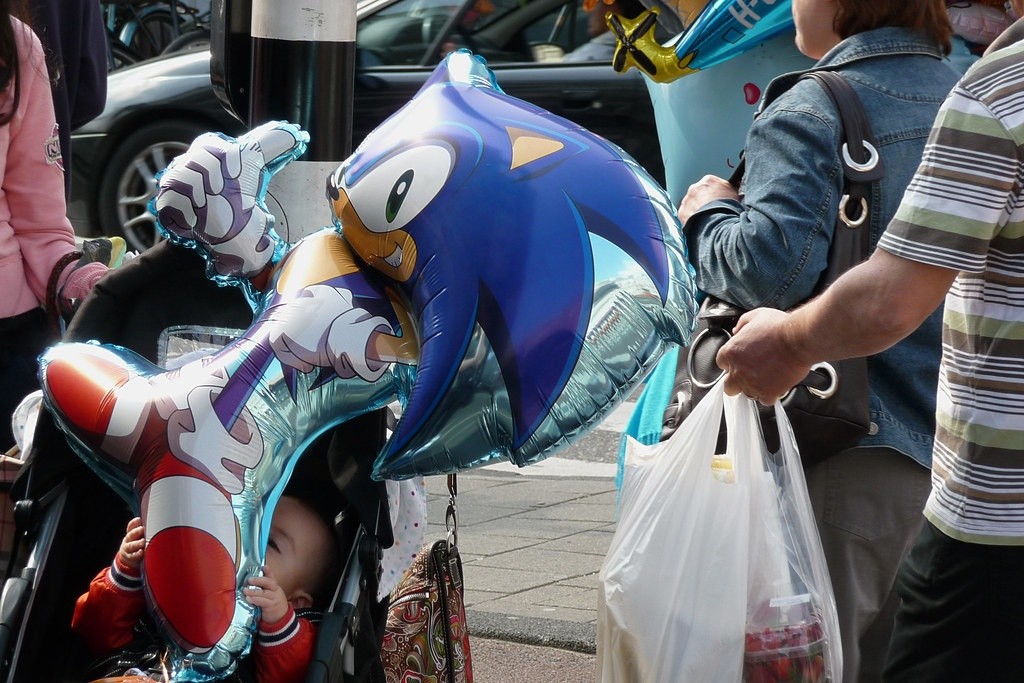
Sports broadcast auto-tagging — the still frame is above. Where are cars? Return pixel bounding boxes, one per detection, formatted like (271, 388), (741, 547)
(64, 0), (670, 274)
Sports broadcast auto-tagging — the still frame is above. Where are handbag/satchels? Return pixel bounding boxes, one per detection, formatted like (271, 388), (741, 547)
(597, 370), (843, 682)
(368, 469), (474, 683)
(658, 69), (882, 473)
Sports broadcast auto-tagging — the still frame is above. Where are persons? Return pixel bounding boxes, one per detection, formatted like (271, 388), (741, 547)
(677, 0), (965, 683)
(1, 0), (114, 575)
(715, 1), (1024, 683)
(69, 469), (337, 682)
(559, 0), (622, 62)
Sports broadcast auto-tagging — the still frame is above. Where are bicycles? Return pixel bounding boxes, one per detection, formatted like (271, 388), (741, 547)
(101, 1), (211, 72)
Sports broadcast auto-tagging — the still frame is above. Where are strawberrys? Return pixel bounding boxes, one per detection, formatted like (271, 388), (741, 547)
(743, 600), (827, 683)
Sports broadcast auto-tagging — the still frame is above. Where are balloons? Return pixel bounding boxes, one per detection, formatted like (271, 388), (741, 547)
(40, 48), (698, 680)
(607, 0), (795, 86)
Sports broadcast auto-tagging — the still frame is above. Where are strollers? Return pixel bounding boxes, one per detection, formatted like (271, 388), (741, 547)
(0, 236), (392, 683)
